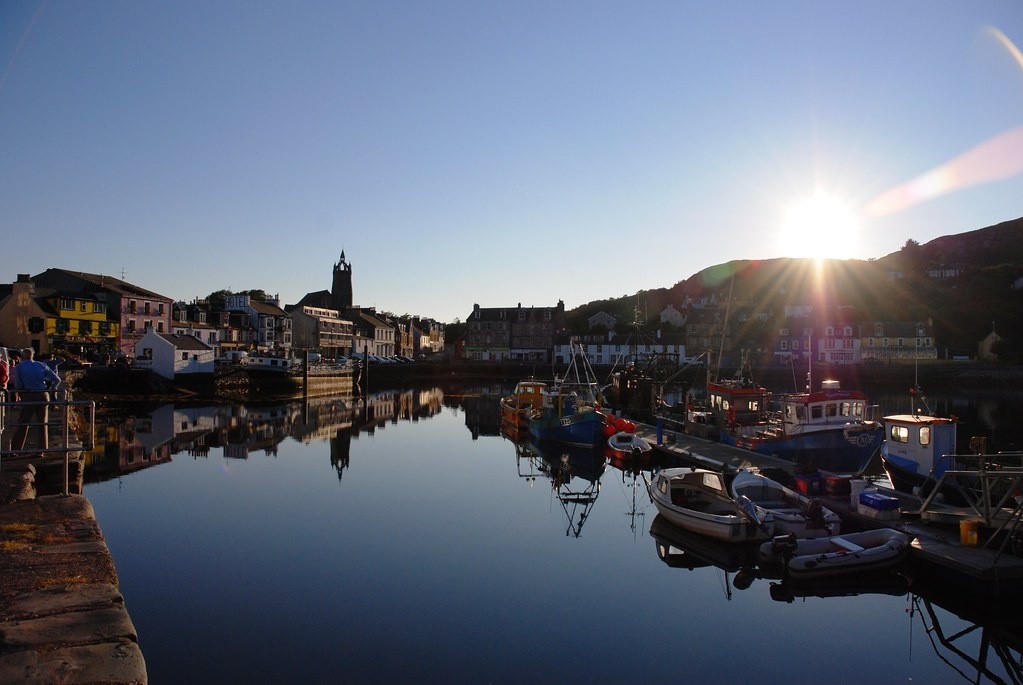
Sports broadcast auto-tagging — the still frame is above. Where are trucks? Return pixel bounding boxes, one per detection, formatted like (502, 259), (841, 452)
(352, 353), (377, 365)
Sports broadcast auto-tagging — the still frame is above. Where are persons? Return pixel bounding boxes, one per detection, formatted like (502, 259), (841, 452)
(0, 353), (21, 434)
(11, 348), (61, 457)
(104, 348), (124, 366)
(43, 352), (66, 409)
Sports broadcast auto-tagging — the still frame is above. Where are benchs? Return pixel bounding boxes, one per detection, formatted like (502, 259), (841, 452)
(751, 499), (789, 507)
(767, 508), (805, 513)
(831, 537), (863, 551)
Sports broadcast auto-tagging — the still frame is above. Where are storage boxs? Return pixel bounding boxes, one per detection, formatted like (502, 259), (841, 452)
(858, 492), (902, 519)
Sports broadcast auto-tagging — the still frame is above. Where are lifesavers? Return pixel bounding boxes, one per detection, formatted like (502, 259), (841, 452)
(720, 406), (736, 425)
(532, 415), (542, 421)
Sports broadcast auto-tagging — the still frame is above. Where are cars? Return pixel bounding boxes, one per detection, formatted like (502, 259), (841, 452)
(325, 355), (363, 364)
(374, 356), (415, 365)
(815, 359), (834, 368)
(784, 359), (802, 367)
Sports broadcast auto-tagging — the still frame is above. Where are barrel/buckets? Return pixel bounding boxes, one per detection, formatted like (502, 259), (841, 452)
(960, 519), (978, 546)
(849, 479), (878, 506)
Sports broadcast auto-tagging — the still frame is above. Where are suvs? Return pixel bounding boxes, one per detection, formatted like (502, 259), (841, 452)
(682, 357), (704, 366)
(863, 357), (883, 366)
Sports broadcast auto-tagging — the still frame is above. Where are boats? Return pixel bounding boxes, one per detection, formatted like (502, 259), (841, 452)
(502, 419), (607, 540)
(649, 512), (761, 574)
(880, 339), (1022, 548)
(731, 467), (841, 543)
(648, 466), (775, 543)
(499, 340), (610, 451)
(761, 528), (908, 580)
(606, 431), (652, 459)
(596, 269), (884, 478)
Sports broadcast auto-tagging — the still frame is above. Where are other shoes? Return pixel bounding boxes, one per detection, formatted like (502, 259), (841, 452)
(36, 451), (45, 457)
(10, 453), (18, 457)
(49, 405), (58, 410)
